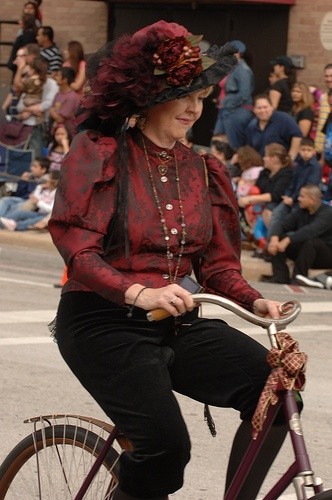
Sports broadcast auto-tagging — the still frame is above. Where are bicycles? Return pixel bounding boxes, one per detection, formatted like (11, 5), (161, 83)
(1, 294), (332, 500)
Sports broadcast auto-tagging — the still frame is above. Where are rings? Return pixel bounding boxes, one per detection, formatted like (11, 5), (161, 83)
(170, 295), (177, 305)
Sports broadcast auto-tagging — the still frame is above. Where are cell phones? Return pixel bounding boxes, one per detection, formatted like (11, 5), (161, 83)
(179, 275), (203, 295)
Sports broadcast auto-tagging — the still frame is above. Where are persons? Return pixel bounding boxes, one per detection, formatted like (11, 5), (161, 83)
(0, 0), (332, 289)
(47, 21), (292, 500)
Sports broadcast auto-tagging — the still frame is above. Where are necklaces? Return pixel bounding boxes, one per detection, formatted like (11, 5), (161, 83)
(156, 154), (171, 175)
(141, 132), (186, 284)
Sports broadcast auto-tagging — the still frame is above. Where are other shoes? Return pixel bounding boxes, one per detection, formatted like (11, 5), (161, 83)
(0, 217), (17, 231)
(295, 274), (324, 288)
(262, 275), (279, 282)
(325, 275), (332, 290)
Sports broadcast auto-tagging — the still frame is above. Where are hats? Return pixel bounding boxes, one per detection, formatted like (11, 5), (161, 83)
(229, 40), (246, 54)
(73, 21), (239, 131)
(269, 56), (292, 66)
